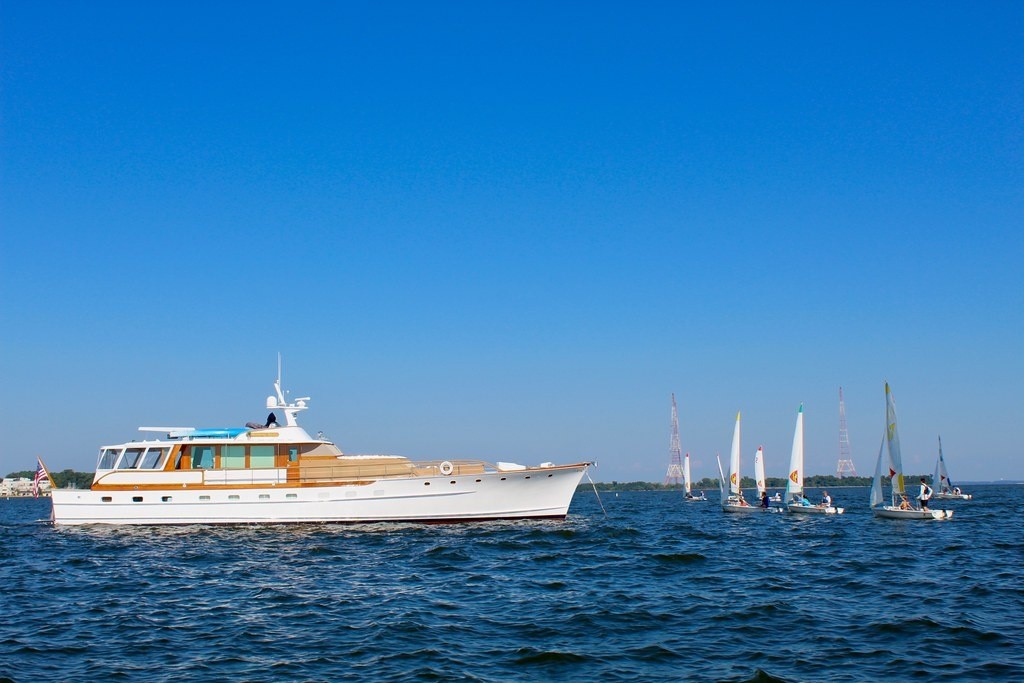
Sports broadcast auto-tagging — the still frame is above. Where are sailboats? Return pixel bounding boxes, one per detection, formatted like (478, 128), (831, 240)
(784, 402), (844, 514)
(716, 411), (783, 513)
(869, 383), (954, 520)
(932, 435), (972, 500)
(755, 445), (805, 507)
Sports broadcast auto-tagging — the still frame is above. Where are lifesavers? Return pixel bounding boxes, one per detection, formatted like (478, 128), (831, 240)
(439, 461), (454, 476)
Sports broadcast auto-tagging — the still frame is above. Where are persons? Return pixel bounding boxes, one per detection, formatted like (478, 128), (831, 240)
(817, 491), (830, 508)
(899, 496), (910, 511)
(952, 485), (960, 495)
(797, 494), (811, 506)
(915, 477), (933, 511)
(760, 492), (771, 508)
(700, 490), (705, 498)
(739, 496), (748, 506)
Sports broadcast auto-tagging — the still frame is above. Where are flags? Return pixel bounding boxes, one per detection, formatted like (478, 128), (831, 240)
(32, 462), (46, 499)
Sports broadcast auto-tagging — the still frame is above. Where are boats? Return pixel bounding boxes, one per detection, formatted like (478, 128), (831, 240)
(0, 478), (53, 497)
(49, 353), (592, 526)
(683, 452), (707, 501)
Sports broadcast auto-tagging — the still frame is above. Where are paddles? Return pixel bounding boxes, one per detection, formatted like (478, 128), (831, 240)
(898, 494), (915, 511)
(737, 495), (752, 507)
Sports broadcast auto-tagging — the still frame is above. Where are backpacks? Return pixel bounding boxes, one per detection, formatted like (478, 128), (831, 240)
(922, 484), (930, 495)
